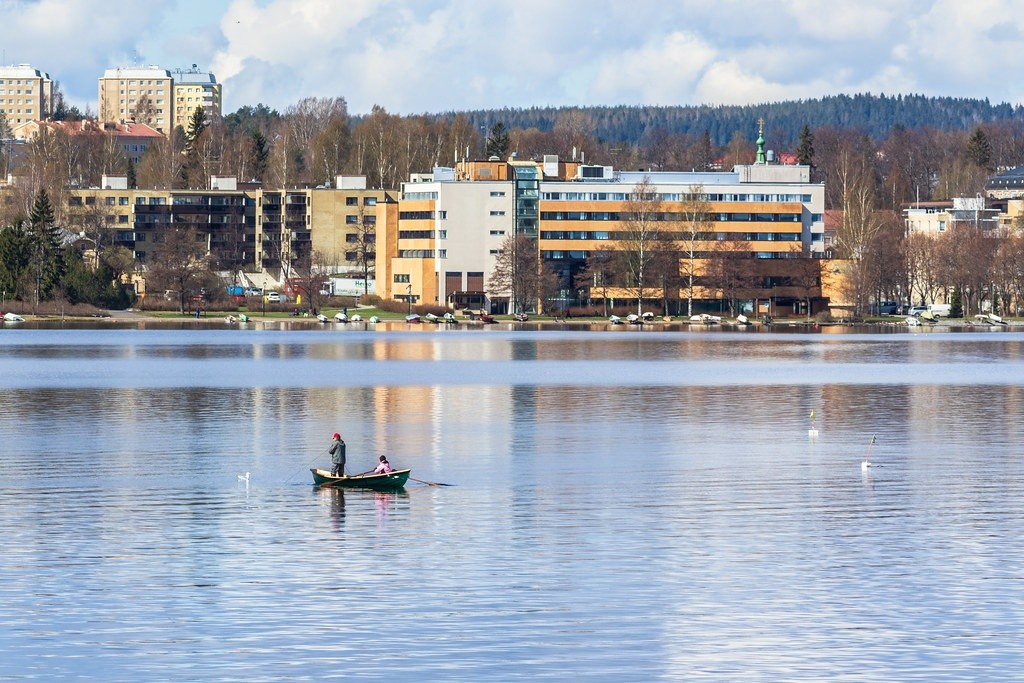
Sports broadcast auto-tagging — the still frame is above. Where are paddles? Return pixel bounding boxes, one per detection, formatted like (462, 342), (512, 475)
(319, 468), (375, 488)
(391, 469), (441, 486)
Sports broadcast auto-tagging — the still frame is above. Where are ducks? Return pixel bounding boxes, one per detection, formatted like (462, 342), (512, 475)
(237, 472), (251, 480)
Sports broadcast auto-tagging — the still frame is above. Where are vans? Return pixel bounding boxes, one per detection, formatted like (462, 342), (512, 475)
(871, 301), (899, 314)
(926, 304), (964, 319)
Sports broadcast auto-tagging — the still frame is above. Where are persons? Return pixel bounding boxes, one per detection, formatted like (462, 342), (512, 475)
(195, 305), (200, 318)
(343, 304), (348, 317)
(699, 314), (711, 321)
(294, 308), (299, 316)
(565, 307), (571, 319)
(313, 308), (318, 316)
(374, 455), (390, 474)
(303, 307), (306, 315)
(470, 311), (474, 320)
(513, 313), (528, 322)
(329, 433), (346, 477)
(478, 313), (486, 321)
(450, 316), (458, 323)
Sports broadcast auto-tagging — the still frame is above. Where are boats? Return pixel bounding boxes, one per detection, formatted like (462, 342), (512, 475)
(479, 313), (494, 322)
(317, 314), (328, 322)
(238, 313), (249, 322)
(626, 314), (639, 324)
(351, 314), (362, 321)
(308, 467), (413, 491)
(444, 313), (455, 323)
(405, 313), (421, 323)
(513, 312), (528, 320)
(426, 312), (438, 323)
(334, 312), (348, 323)
(369, 315), (380, 323)
(736, 313), (748, 323)
(224, 314), (237, 323)
(5, 316), (25, 326)
(642, 312), (654, 319)
(610, 314), (620, 324)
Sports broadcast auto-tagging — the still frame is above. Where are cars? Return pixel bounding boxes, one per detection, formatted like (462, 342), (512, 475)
(249, 289), (262, 297)
(263, 291), (281, 303)
(895, 305), (910, 316)
(164, 290), (179, 301)
(244, 289), (249, 297)
(907, 306), (927, 317)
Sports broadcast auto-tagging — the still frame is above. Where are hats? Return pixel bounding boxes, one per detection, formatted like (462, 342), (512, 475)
(333, 433), (340, 439)
